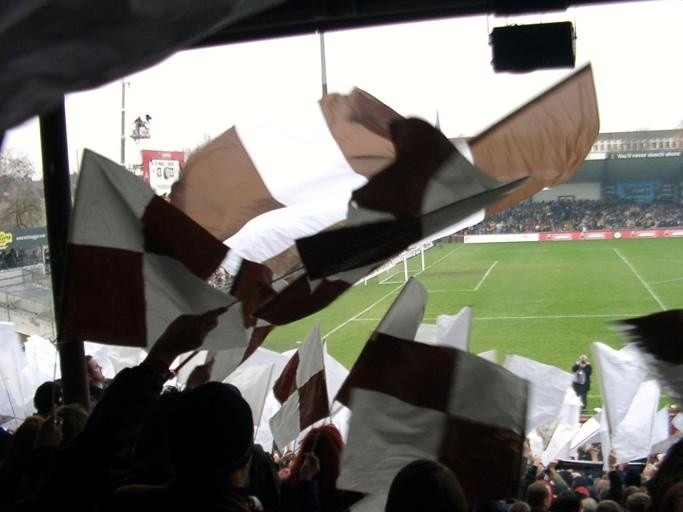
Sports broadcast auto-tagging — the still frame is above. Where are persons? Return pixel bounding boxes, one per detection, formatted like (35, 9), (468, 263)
(1, 244), (49, 273)
(1, 353), (683, 511)
(465, 199), (683, 236)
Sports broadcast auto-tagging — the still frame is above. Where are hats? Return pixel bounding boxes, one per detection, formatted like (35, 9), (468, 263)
(168, 381), (254, 479)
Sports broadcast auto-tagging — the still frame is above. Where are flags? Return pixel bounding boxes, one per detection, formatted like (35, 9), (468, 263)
(296, 118), (531, 283)
(320, 62), (600, 223)
